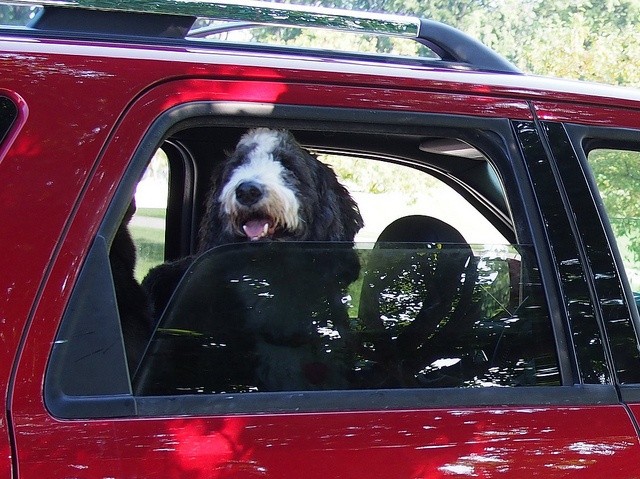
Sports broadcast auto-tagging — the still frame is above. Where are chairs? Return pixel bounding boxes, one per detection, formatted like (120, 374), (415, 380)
(351, 215), (493, 391)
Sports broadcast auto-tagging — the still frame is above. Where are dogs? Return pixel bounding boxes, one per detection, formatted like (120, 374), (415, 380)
(141, 126), (363, 392)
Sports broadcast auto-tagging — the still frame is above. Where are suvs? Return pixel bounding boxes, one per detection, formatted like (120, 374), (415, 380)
(0, 0), (639, 477)
